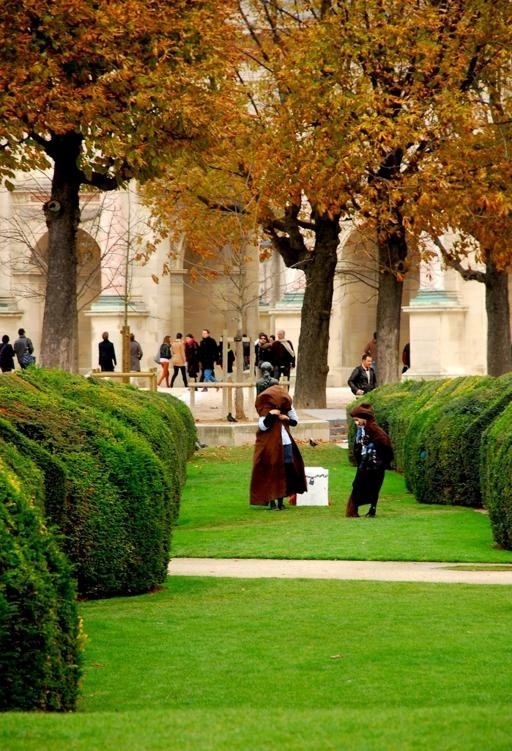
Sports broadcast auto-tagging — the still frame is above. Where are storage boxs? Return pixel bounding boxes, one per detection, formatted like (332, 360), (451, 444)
(296, 467), (329, 507)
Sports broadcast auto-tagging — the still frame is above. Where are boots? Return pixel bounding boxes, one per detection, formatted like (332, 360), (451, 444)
(365, 508), (376, 518)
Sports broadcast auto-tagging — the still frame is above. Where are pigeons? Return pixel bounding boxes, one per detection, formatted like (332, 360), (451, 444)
(227, 413), (238, 424)
(197, 440), (208, 448)
(310, 440), (318, 448)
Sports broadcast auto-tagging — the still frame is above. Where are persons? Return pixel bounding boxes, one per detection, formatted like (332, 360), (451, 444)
(129, 333), (143, 387)
(347, 353), (377, 396)
(401, 343), (410, 374)
(13, 328), (34, 370)
(364, 332), (377, 371)
(156, 329), (297, 395)
(99, 332), (117, 371)
(346, 404), (394, 518)
(250, 385), (307, 511)
(0, 334), (15, 372)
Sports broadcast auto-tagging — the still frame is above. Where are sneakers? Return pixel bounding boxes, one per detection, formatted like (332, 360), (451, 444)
(271, 505), (289, 511)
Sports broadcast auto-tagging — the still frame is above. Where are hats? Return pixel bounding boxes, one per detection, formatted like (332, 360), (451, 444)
(350, 404), (375, 420)
